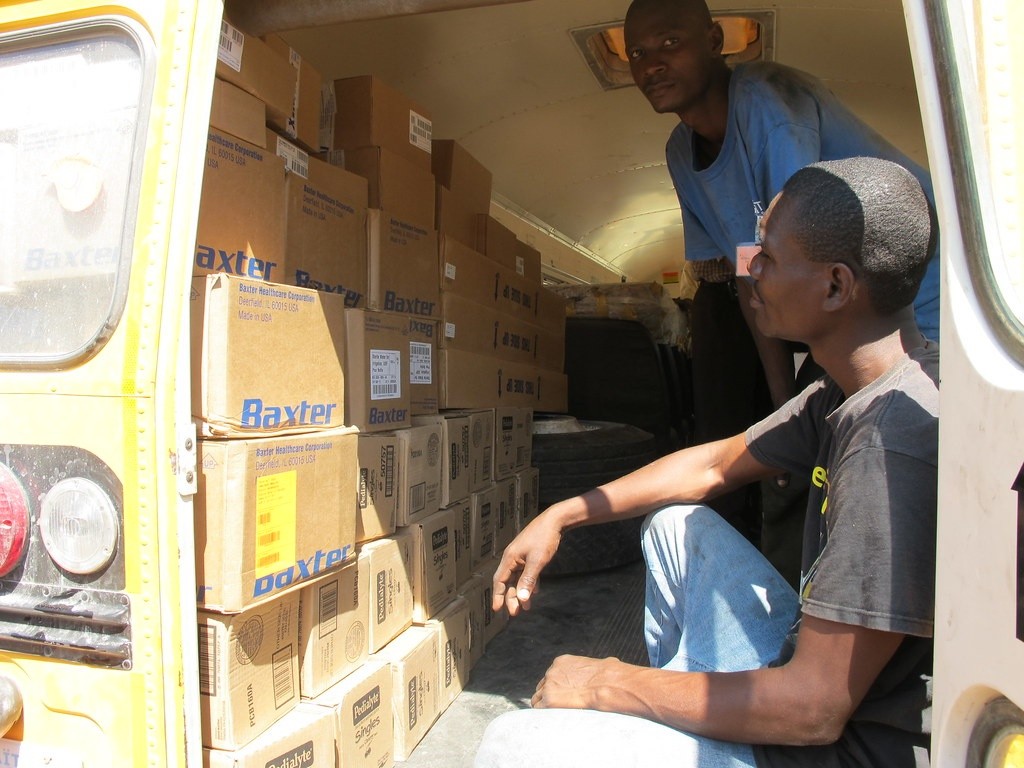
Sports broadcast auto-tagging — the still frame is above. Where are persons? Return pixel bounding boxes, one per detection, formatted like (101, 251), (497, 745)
(622, 0), (941, 594)
(466, 151), (942, 768)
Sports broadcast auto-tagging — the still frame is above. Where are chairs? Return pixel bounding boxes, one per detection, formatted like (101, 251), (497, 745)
(568, 321), (695, 453)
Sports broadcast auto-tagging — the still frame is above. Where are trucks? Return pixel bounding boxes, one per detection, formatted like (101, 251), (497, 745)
(3, 0), (1024, 768)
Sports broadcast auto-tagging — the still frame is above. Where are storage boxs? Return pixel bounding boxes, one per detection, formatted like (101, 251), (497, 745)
(189, 15), (568, 768)
